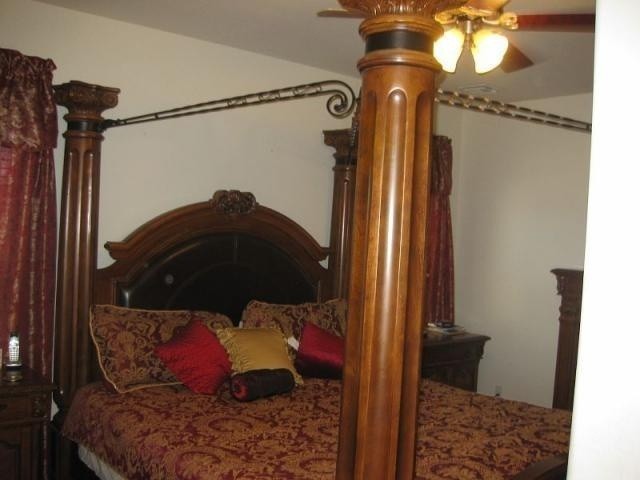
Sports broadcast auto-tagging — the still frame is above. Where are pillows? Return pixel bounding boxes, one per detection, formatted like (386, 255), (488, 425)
(89, 298), (347, 404)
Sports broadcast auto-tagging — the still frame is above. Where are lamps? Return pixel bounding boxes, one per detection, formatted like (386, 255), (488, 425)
(434, 7), (518, 73)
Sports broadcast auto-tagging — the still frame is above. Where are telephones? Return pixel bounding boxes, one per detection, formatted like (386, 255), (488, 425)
(3, 332), (22, 367)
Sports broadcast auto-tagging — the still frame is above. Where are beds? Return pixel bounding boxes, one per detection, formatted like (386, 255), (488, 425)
(48, 190), (572, 480)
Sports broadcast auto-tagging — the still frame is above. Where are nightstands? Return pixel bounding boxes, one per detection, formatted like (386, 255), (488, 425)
(2, 364), (59, 479)
(420, 328), (491, 393)
